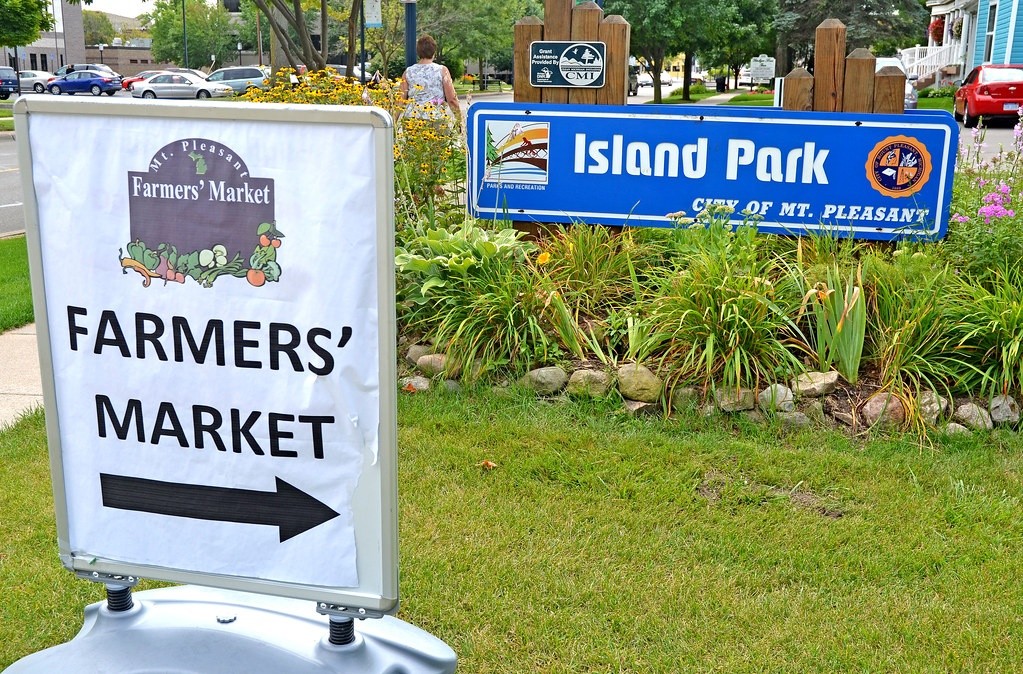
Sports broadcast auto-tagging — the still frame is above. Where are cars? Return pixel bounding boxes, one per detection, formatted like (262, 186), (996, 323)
(462, 74), (479, 84)
(119, 69), (209, 92)
(739, 72), (755, 86)
(262, 64), (372, 87)
(15, 71), (63, 94)
(637, 70), (674, 88)
(629, 65), (639, 97)
(131, 72), (233, 99)
(691, 77), (705, 85)
(954, 64), (1023, 128)
(47, 70), (121, 96)
(875, 57), (919, 109)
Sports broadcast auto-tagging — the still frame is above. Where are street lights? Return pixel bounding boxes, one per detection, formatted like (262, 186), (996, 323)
(98, 44), (103, 63)
(237, 41), (243, 66)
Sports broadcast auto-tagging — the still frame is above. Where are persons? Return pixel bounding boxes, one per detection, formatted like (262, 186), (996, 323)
(393, 35), (462, 134)
(66, 64), (75, 74)
(291, 66), (305, 89)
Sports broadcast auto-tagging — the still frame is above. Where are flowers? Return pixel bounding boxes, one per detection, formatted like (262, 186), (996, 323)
(948, 17), (963, 40)
(928, 18), (944, 45)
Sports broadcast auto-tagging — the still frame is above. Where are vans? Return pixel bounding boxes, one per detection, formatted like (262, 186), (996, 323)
(207, 66), (269, 95)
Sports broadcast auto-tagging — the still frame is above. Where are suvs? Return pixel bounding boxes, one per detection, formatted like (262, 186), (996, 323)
(54, 63), (114, 73)
(0, 66), (19, 101)
